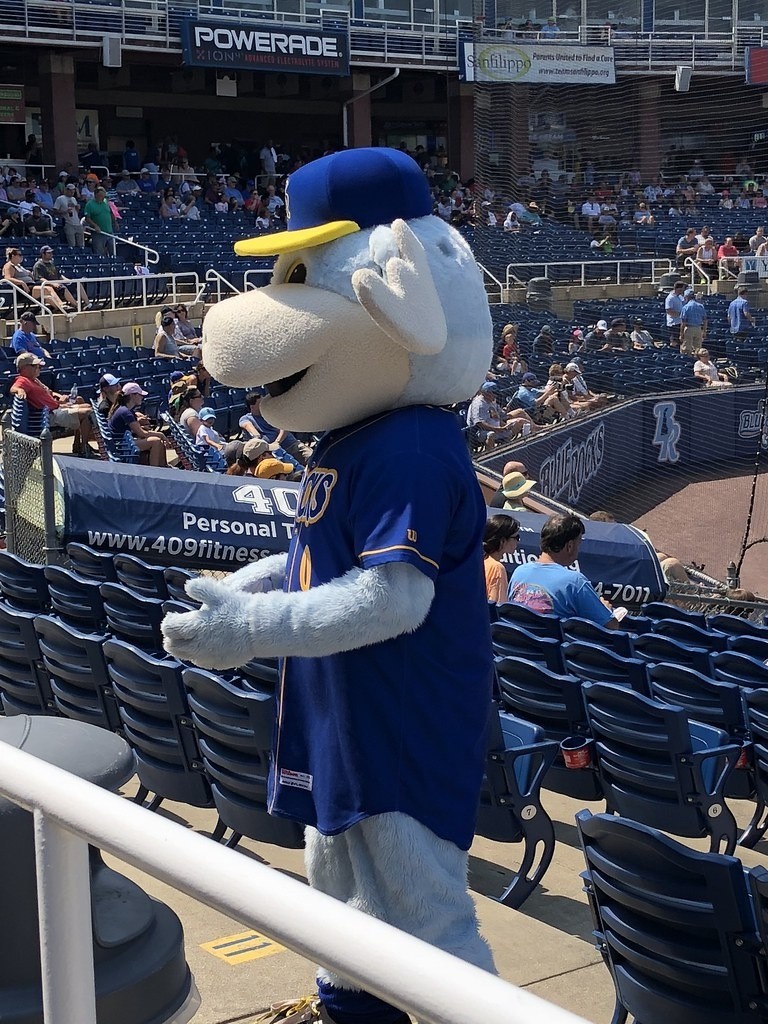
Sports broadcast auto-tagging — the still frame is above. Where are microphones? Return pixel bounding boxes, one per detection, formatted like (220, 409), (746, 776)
(554, 377), (563, 382)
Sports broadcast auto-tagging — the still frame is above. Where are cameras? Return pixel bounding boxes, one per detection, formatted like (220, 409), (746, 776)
(554, 383), (574, 391)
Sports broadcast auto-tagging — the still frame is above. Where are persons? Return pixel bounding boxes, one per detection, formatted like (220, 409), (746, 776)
(24, 134), (36, 159)
(466, 317), (661, 451)
(374, 137), (768, 277)
(482, 461), (757, 631)
(499, 16), (560, 38)
(665, 225), (768, 390)
(0, 140), (202, 313)
(202, 138), (345, 230)
(9, 304), (323, 481)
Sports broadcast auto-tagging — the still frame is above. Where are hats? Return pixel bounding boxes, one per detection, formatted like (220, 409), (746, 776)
(121, 382), (148, 395)
(21, 312), (41, 325)
(160, 306), (179, 315)
(99, 373), (121, 389)
(0, 162), (730, 397)
(243, 438), (280, 462)
(16, 353), (45, 369)
(169, 381), (192, 403)
(502, 471), (538, 499)
(254, 458), (294, 480)
(199, 407), (216, 420)
(39, 245), (54, 254)
(170, 370), (191, 383)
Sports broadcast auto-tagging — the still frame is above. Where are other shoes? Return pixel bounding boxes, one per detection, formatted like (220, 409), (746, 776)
(81, 302), (104, 311)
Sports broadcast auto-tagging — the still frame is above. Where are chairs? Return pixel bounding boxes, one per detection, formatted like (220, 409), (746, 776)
(0, 192), (768, 1024)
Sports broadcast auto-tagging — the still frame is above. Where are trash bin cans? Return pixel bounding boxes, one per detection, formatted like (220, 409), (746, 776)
(527, 276), (553, 313)
(658, 272), (682, 298)
(0, 713), (202, 1024)
(738, 269), (761, 308)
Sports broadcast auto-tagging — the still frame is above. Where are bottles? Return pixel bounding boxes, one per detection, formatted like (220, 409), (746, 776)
(70, 382), (78, 403)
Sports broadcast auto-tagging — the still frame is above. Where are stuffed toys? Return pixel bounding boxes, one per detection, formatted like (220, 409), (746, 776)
(201, 144), (499, 1024)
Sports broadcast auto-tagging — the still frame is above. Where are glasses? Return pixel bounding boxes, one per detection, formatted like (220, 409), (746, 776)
(510, 534), (520, 542)
(521, 469), (529, 477)
(265, 450), (272, 455)
(12, 253), (23, 256)
(177, 309), (185, 314)
(26, 364), (38, 367)
(191, 394), (203, 399)
